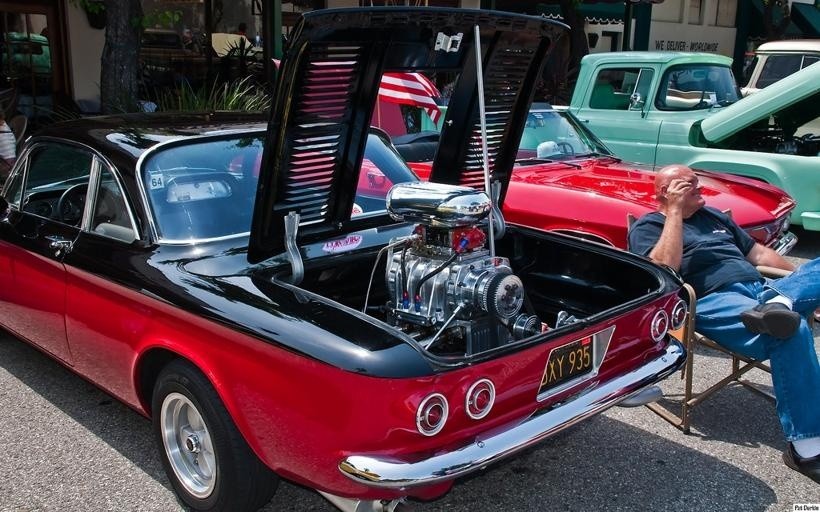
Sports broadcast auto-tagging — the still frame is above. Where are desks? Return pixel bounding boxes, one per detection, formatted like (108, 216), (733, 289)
(0, 117), (17, 183)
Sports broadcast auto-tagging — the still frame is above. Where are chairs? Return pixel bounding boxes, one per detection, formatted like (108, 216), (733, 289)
(536, 141), (564, 160)
(7, 115), (28, 158)
(590, 79), (615, 109)
(627, 215), (811, 433)
(0, 86), (18, 121)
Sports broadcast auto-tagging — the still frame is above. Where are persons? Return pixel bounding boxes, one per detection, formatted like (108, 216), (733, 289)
(625, 164), (820, 481)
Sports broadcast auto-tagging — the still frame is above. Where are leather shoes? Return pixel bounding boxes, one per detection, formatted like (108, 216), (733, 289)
(781, 440), (819, 487)
(738, 300), (803, 340)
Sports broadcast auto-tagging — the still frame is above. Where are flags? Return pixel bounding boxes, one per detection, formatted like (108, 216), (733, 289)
(380, 71), (443, 128)
